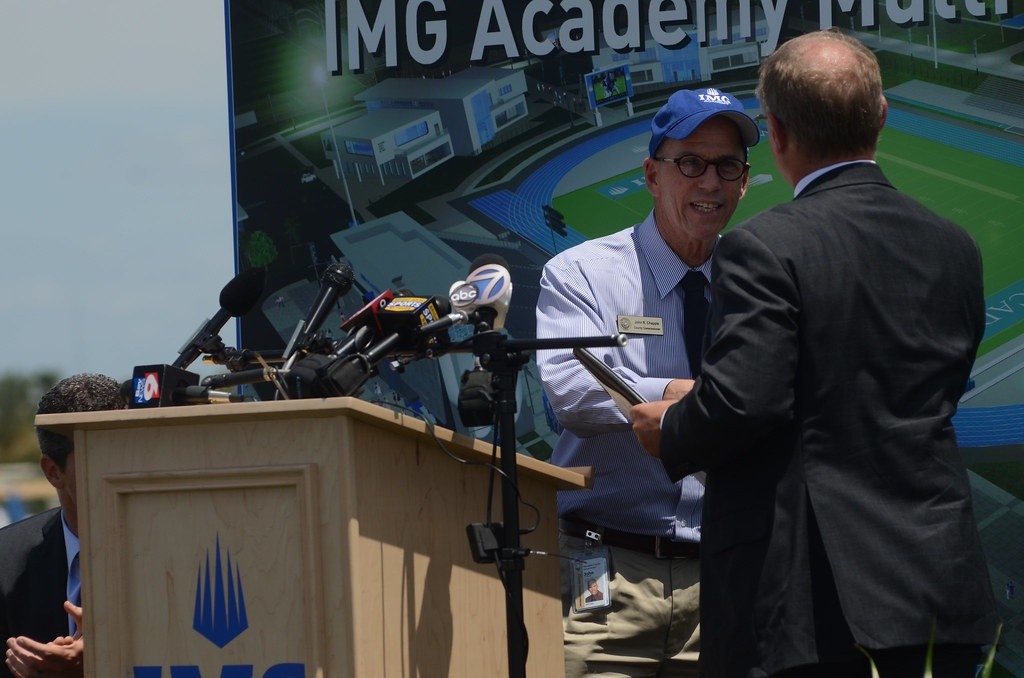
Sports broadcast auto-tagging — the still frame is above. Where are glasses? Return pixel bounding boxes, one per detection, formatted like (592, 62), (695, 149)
(653, 155), (751, 181)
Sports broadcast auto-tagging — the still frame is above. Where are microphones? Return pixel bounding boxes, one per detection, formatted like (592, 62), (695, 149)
(118, 253), (514, 425)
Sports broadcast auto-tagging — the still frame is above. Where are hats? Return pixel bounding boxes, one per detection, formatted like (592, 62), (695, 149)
(650, 86), (759, 155)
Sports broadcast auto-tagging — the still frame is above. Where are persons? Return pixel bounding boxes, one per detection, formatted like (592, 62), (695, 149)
(338, 312), (347, 326)
(373, 381), (402, 407)
(629, 29), (998, 678)
(602, 71), (620, 97)
(0, 374), (127, 678)
(585, 579), (604, 602)
(535, 87), (761, 678)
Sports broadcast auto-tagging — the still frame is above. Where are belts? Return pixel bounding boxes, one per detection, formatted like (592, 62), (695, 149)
(563, 517), (703, 559)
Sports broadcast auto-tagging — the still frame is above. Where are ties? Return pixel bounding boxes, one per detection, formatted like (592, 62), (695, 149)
(680, 270), (712, 376)
